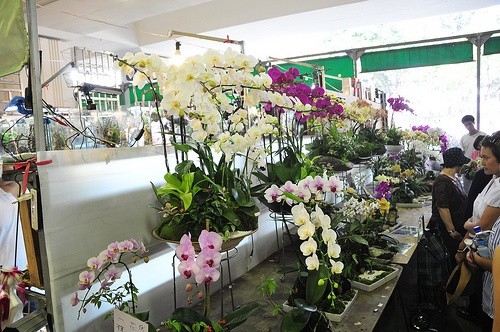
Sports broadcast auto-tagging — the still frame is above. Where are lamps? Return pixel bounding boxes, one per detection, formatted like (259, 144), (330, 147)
(175, 42), (181, 55)
(4, 97), (24, 112)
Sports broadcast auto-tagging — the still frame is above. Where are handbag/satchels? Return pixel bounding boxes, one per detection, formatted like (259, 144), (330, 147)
(445, 262), (473, 305)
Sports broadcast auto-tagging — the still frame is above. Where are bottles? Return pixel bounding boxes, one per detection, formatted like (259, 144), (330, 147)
(473, 226), (492, 258)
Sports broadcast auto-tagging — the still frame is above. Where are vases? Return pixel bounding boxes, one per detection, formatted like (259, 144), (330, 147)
(258, 193), (315, 212)
(396, 199), (425, 207)
(152, 227), (257, 255)
(347, 265), (399, 291)
(389, 221), (402, 231)
(384, 145), (404, 153)
(368, 247), (400, 263)
(283, 288), (358, 322)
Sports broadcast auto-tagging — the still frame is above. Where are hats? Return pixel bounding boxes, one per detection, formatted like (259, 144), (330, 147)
(440, 147), (471, 166)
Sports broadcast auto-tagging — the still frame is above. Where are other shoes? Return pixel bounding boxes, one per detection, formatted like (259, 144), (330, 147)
(456, 306), (479, 320)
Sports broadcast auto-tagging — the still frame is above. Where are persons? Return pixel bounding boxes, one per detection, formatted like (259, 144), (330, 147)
(0, 178), (28, 273)
(432, 147), (472, 272)
(460, 115), (487, 160)
(481, 215), (500, 332)
(455, 130), (500, 321)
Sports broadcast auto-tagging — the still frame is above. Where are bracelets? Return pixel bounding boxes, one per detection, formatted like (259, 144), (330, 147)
(447, 228), (455, 235)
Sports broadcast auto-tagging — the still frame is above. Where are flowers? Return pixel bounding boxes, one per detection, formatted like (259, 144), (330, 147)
(70, 47), (448, 332)
